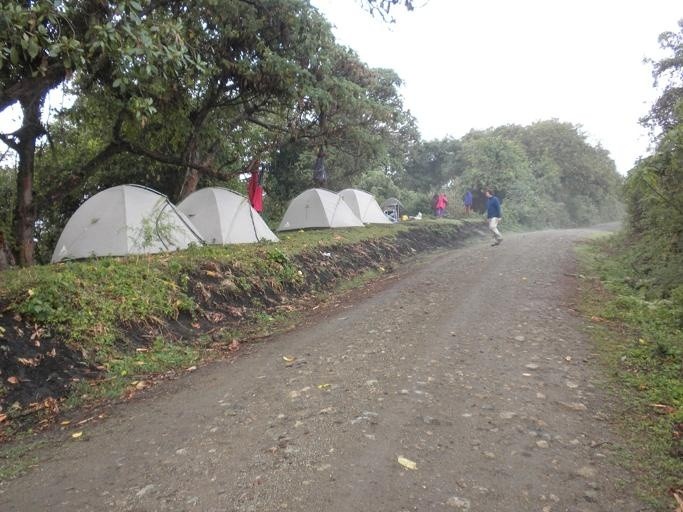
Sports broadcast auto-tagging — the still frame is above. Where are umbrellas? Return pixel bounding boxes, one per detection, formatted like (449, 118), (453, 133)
(246, 159), (263, 213)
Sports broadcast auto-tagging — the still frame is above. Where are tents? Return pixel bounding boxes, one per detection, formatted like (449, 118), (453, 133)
(49, 183), (207, 265)
(173, 186), (280, 245)
(379, 197), (408, 223)
(336, 188), (391, 224)
(275, 187), (365, 231)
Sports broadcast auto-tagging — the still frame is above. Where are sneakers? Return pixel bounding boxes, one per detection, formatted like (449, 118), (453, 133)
(492, 242), (499, 246)
(496, 238), (503, 244)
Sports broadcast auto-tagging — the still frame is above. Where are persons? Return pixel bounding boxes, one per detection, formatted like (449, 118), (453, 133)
(485, 189), (503, 246)
(414, 210), (422, 220)
(464, 190), (471, 218)
(433, 191), (448, 217)
